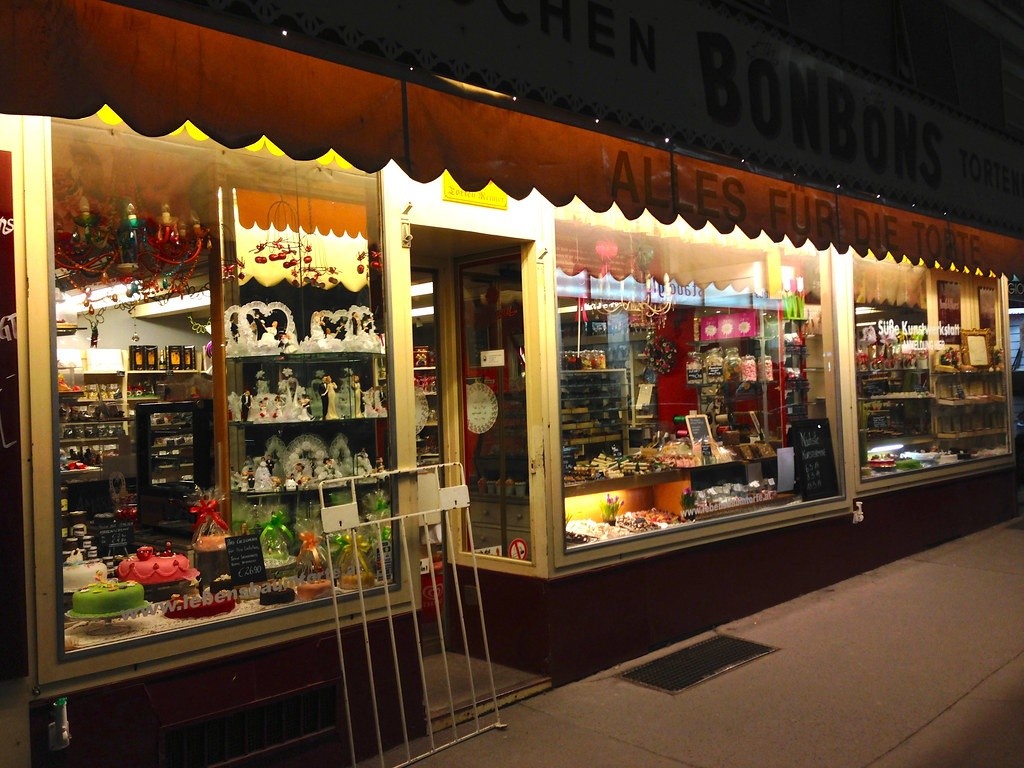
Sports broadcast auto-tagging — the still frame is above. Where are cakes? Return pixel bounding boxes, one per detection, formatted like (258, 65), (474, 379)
(165, 573), (241, 620)
(259, 585), (296, 605)
(193, 533), (232, 551)
(115, 541), (190, 585)
(68, 578), (148, 618)
(339, 570), (375, 590)
(62, 561), (107, 593)
(297, 578), (332, 600)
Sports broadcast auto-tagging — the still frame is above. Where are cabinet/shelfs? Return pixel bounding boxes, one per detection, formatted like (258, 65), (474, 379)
(936, 364), (1007, 439)
(858, 368), (933, 445)
(59, 369), (629, 541)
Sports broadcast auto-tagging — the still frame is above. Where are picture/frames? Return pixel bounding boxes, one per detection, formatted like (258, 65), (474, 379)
(685, 415), (713, 447)
(959, 328), (994, 369)
